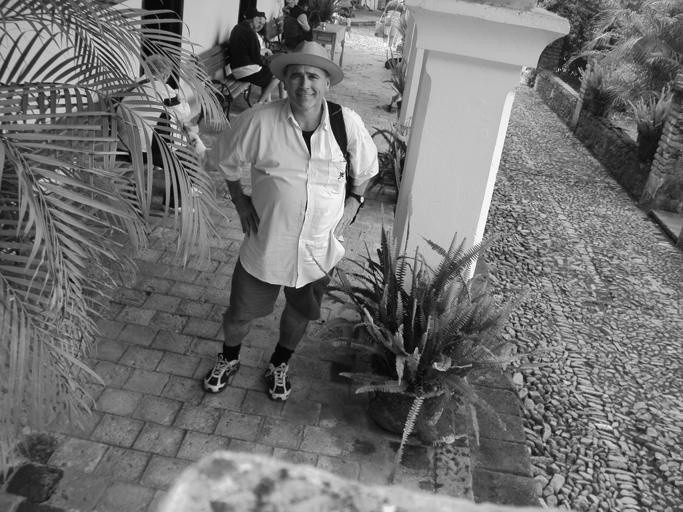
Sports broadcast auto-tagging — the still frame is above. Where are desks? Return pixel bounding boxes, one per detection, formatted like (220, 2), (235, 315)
(310, 21), (347, 69)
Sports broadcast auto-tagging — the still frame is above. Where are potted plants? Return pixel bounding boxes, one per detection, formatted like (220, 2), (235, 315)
(575, 57), (621, 118)
(625, 84), (673, 163)
(297, 230), (535, 484)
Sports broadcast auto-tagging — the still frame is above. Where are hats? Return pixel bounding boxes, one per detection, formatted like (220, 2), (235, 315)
(242, 7), (264, 20)
(269, 41), (346, 88)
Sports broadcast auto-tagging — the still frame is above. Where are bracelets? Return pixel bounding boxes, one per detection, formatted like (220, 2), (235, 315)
(349, 192), (365, 203)
(229, 191), (245, 204)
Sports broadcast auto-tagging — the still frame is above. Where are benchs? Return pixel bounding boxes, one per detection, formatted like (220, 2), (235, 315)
(259, 16), (291, 57)
(9, 90), (155, 249)
(190, 32), (289, 125)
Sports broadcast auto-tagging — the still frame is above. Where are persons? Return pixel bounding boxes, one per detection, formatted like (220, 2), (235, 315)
(228, 1), (321, 105)
(201, 41), (380, 403)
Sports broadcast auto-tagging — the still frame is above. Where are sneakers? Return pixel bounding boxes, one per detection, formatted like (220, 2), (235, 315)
(202, 354), (240, 393)
(264, 360), (292, 402)
